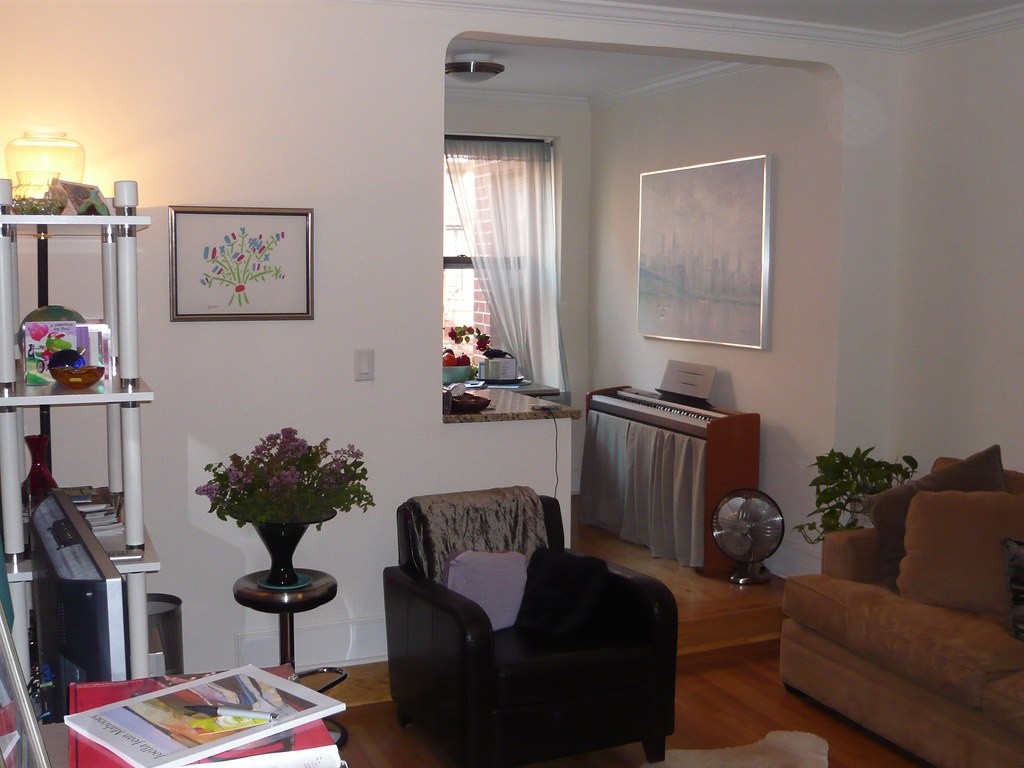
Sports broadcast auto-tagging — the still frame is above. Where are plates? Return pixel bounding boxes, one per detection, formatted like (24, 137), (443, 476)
(474, 375), (524, 384)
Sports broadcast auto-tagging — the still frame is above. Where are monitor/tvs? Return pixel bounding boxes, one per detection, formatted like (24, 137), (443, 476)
(29, 487), (145, 725)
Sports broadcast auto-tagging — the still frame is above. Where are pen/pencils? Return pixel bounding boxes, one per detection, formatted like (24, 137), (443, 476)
(183, 702), (279, 721)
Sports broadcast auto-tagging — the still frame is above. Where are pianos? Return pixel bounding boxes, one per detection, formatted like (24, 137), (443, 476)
(583, 385), (762, 574)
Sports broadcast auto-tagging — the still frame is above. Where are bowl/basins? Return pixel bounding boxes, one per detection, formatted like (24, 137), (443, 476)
(48, 366), (105, 390)
(443, 365), (471, 385)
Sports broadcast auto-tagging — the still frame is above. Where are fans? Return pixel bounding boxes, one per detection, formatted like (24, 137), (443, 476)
(711, 487), (786, 585)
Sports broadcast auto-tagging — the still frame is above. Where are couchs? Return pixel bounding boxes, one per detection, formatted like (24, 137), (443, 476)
(782, 456), (1024, 768)
(385, 486), (678, 768)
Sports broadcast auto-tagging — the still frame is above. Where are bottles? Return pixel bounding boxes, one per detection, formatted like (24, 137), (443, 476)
(12, 169), (69, 216)
(4, 128), (86, 216)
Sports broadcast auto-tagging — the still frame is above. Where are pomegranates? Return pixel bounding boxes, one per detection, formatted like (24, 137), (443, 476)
(443, 347), (470, 367)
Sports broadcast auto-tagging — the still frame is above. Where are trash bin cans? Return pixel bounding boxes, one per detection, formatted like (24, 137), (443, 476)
(144, 592), (186, 675)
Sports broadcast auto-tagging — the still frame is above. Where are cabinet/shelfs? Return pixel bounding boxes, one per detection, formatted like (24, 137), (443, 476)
(0, 179), (161, 708)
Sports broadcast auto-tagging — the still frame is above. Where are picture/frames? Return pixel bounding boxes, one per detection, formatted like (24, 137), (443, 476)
(636, 153), (773, 350)
(168, 206), (315, 323)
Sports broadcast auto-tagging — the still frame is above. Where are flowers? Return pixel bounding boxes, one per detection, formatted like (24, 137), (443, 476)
(194, 426), (376, 532)
(448, 326), (492, 352)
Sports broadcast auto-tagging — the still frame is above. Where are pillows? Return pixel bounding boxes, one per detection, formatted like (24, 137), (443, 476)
(862, 443), (1009, 590)
(897, 490), (1024, 613)
(638, 730), (830, 768)
(515, 547), (607, 642)
(444, 549), (527, 633)
(1001, 537), (1024, 640)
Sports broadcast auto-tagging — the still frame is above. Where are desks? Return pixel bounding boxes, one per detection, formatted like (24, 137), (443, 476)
(234, 567), (348, 750)
(488, 378), (560, 399)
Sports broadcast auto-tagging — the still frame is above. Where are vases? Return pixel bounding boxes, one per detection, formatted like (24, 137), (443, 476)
(228, 501), (337, 585)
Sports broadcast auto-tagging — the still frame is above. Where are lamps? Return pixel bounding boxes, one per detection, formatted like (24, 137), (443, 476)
(445, 53), (505, 84)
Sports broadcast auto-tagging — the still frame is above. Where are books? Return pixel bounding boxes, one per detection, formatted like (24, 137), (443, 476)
(68, 663), (339, 768)
(63, 665), (346, 768)
(22, 485), (125, 539)
